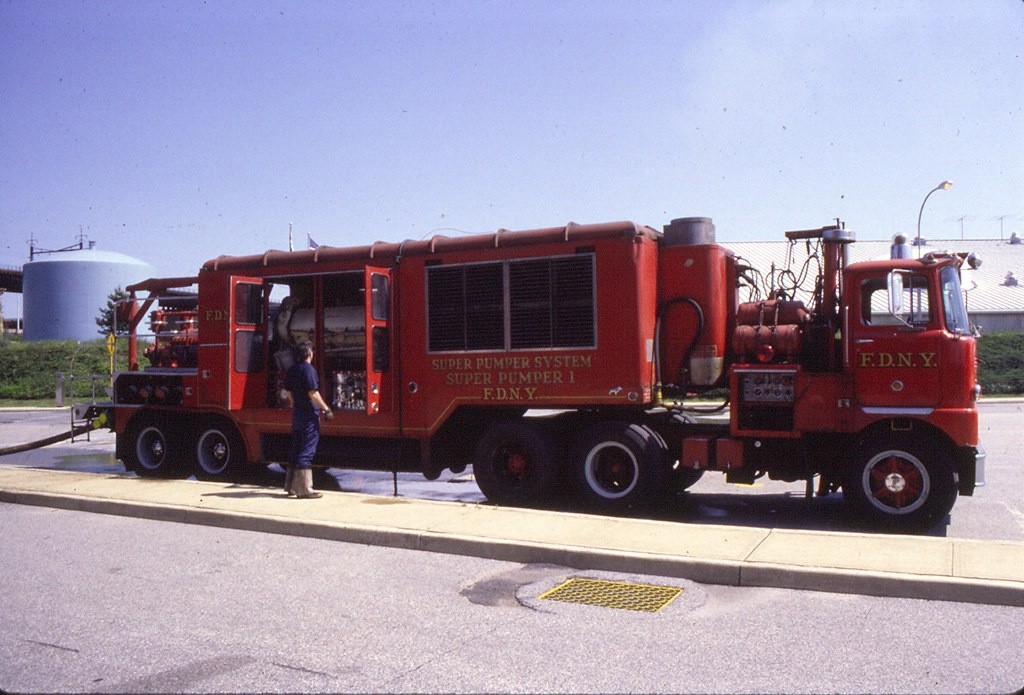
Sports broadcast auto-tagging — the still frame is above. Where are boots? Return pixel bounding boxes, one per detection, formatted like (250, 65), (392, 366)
(284, 466), (322, 499)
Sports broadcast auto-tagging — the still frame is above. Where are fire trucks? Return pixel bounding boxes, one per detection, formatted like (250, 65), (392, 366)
(66, 178), (985, 528)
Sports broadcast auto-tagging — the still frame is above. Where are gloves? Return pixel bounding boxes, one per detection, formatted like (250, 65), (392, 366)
(325, 408), (334, 421)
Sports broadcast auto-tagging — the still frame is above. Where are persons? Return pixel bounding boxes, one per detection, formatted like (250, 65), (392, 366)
(283, 341), (334, 498)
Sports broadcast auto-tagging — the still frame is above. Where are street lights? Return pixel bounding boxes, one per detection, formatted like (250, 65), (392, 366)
(915, 180), (953, 320)
